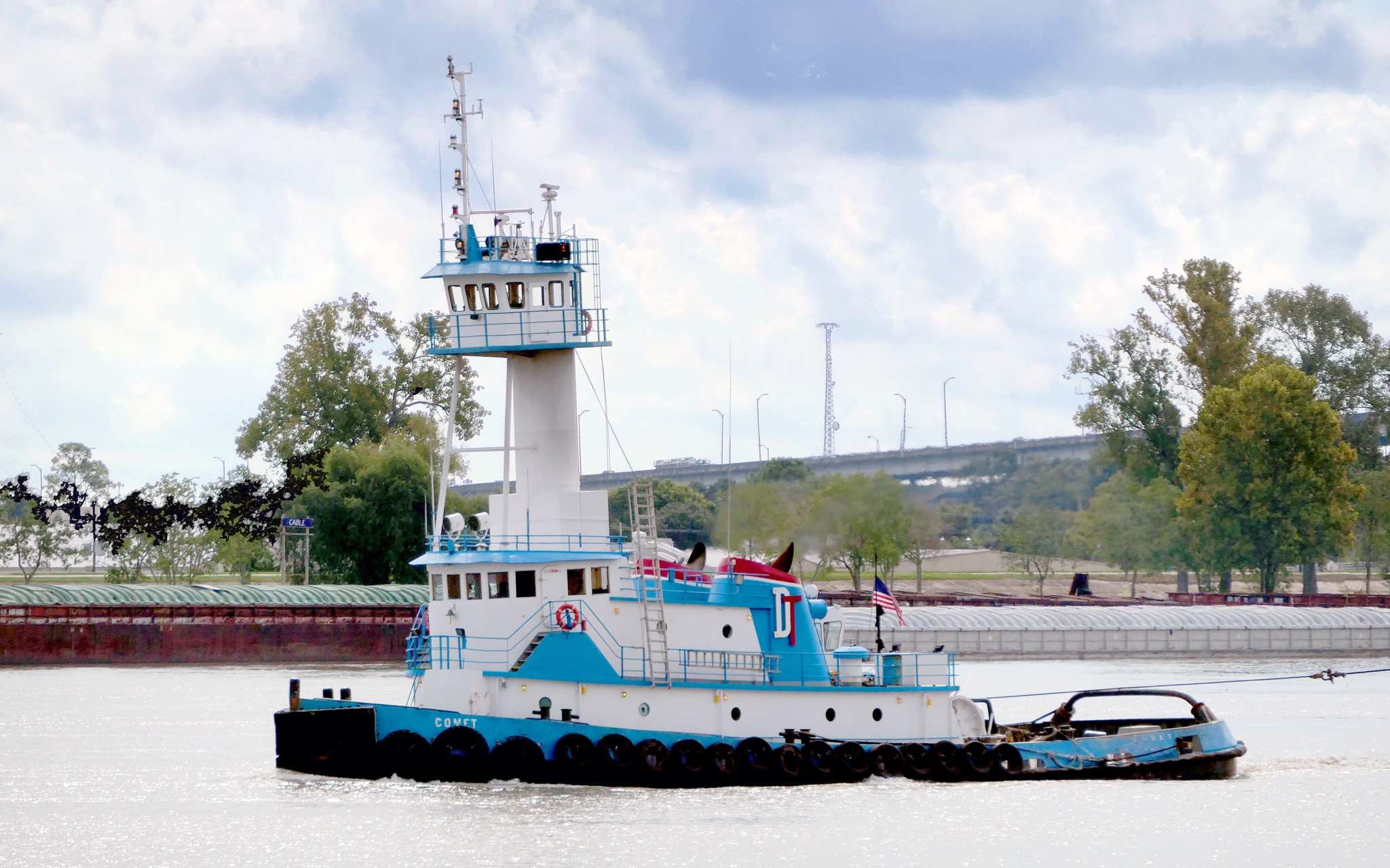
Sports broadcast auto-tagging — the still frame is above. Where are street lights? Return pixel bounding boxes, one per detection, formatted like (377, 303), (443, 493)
(757, 393), (773, 460)
(757, 444), (771, 461)
(894, 394), (906, 449)
(868, 436), (880, 451)
(712, 409), (724, 464)
(944, 377), (957, 446)
(577, 410), (591, 476)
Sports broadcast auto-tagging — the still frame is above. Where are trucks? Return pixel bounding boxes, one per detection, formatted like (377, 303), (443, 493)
(654, 457), (710, 469)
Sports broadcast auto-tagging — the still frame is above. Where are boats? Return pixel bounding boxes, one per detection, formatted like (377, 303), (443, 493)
(270, 55), (1251, 784)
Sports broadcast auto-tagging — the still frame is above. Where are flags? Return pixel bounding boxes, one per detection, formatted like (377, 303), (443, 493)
(872, 575), (906, 626)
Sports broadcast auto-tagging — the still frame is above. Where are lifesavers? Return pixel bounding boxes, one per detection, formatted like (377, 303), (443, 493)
(581, 308), (592, 336)
(555, 603), (580, 629)
